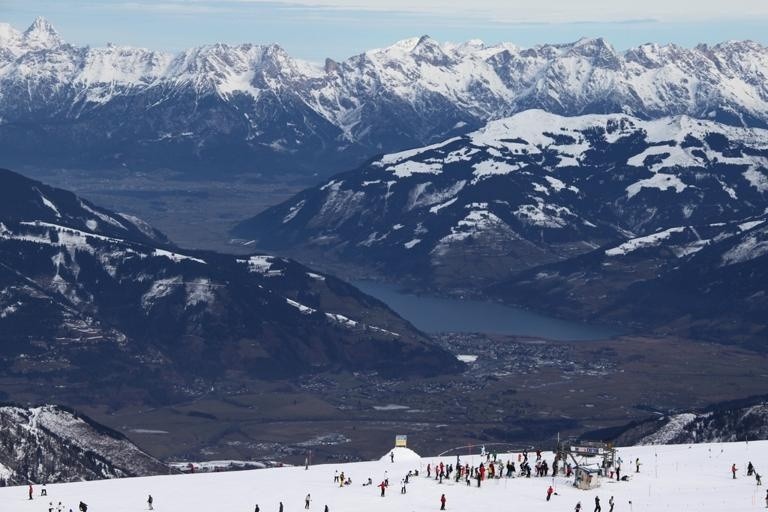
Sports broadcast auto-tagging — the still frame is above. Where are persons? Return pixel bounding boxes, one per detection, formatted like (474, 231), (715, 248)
(255, 504), (260, 511)
(49, 501), (88, 512)
(405, 470), (418, 482)
(440, 494), (446, 510)
(400, 479), (406, 494)
(575, 501), (581, 511)
(427, 450), (641, 487)
(305, 493), (312, 509)
(334, 470), (352, 487)
(279, 502), (283, 511)
(304, 455), (309, 469)
(546, 486), (553, 500)
(384, 471), (389, 486)
(765, 489), (768, 508)
(610, 497), (615, 511)
(324, 505), (328, 511)
(40, 483), (47, 496)
(390, 452), (394, 463)
(732, 461), (761, 486)
(147, 494), (153, 510)
(29, 484), (33, 500)
(594, 496), (602, 511)
(380, 481), (385, 496)
(363, 478), (373, 486)
